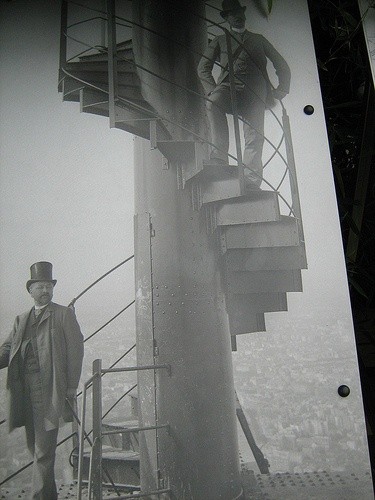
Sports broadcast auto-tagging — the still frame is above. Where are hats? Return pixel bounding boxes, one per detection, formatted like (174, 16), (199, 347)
(219, 0), (247, 18)
(26, 261), (57, 293)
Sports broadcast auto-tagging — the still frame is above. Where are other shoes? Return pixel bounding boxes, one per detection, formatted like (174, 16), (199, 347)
(32, 487), (56, 499)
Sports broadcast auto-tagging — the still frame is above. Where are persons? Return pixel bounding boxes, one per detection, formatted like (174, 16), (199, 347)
(0, 261), (84, 500)
(196, 0), (291, 192)
